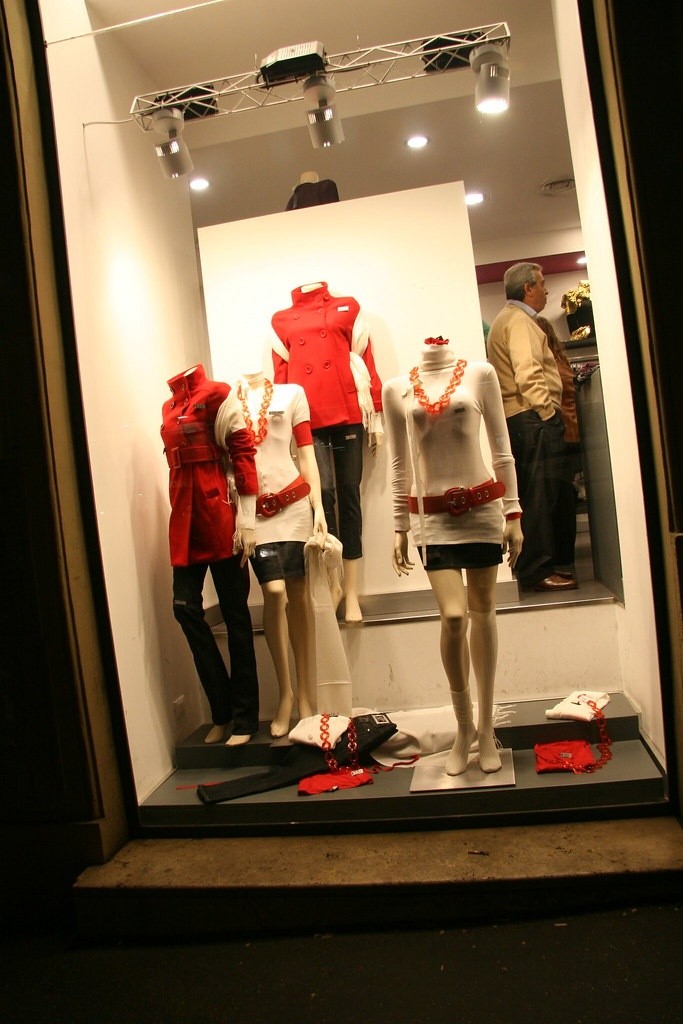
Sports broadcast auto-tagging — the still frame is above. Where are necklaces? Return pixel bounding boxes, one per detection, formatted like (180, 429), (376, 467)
(234, 378), (273, 446)
(407, 359), (468, 415)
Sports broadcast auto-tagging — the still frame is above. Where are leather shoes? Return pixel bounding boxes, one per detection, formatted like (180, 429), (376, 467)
(521, 574), (578, 592)
(555, 570), (572, 579)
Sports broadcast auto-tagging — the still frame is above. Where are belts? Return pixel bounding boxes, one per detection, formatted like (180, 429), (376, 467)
(408, 478), (506, 517)
(256, 474), (311, 518)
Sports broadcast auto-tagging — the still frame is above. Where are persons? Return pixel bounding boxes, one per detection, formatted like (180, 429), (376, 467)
(485, 260), (580, 585)
(384, 333), (523, 775)
(271, 280), (384, 622)
(171, 364), (258, 746)
(224, 363), (328, 735)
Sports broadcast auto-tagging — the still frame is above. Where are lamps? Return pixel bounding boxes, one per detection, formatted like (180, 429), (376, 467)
(470, 47), (512, 117)
(300, 74), (347, 148)
(153, 107), (194, 178)
